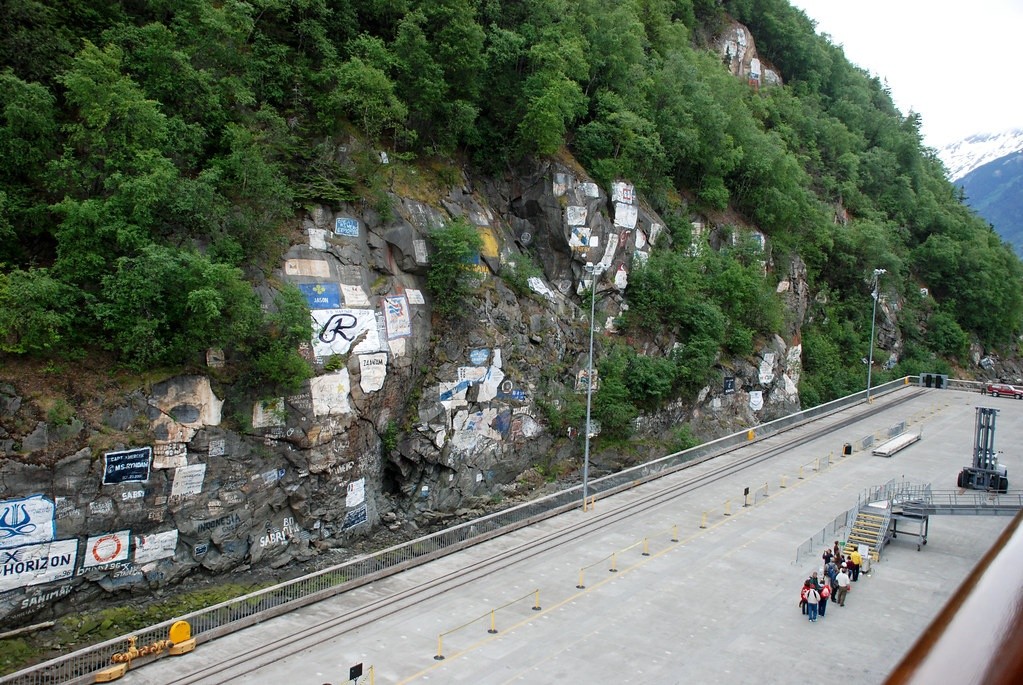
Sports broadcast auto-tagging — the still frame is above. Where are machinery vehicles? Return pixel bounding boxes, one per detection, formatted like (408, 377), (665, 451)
(957, 407), (1009, 494)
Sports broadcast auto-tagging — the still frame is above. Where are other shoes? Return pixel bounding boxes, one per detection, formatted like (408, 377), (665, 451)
(840, 604), (846, 607)
(832, 599), (837, 602)
(813, 619), (818, 622)
(818, 615), (825, 618)
(809, 620), (812, 622)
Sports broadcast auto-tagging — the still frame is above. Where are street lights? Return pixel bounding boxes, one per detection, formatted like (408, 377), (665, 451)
(867, 269), (886, 402)
(581, 261), (610, 511)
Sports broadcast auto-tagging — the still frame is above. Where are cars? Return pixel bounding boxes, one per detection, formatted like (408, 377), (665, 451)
(987, 384), (1023, 400)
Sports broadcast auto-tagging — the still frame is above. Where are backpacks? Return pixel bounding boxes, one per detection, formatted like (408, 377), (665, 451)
(801, 588), (808, 600)
(827, 563), (837, 578)
(820, 586), (830, 598)
(803, 578), (811, 587)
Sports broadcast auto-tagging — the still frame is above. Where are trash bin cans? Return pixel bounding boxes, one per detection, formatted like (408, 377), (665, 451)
(844, 446), (852, 455)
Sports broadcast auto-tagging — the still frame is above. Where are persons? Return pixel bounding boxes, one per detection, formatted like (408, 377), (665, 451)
(981, 381), (986, 394)
(799, 541), (862, 623)
